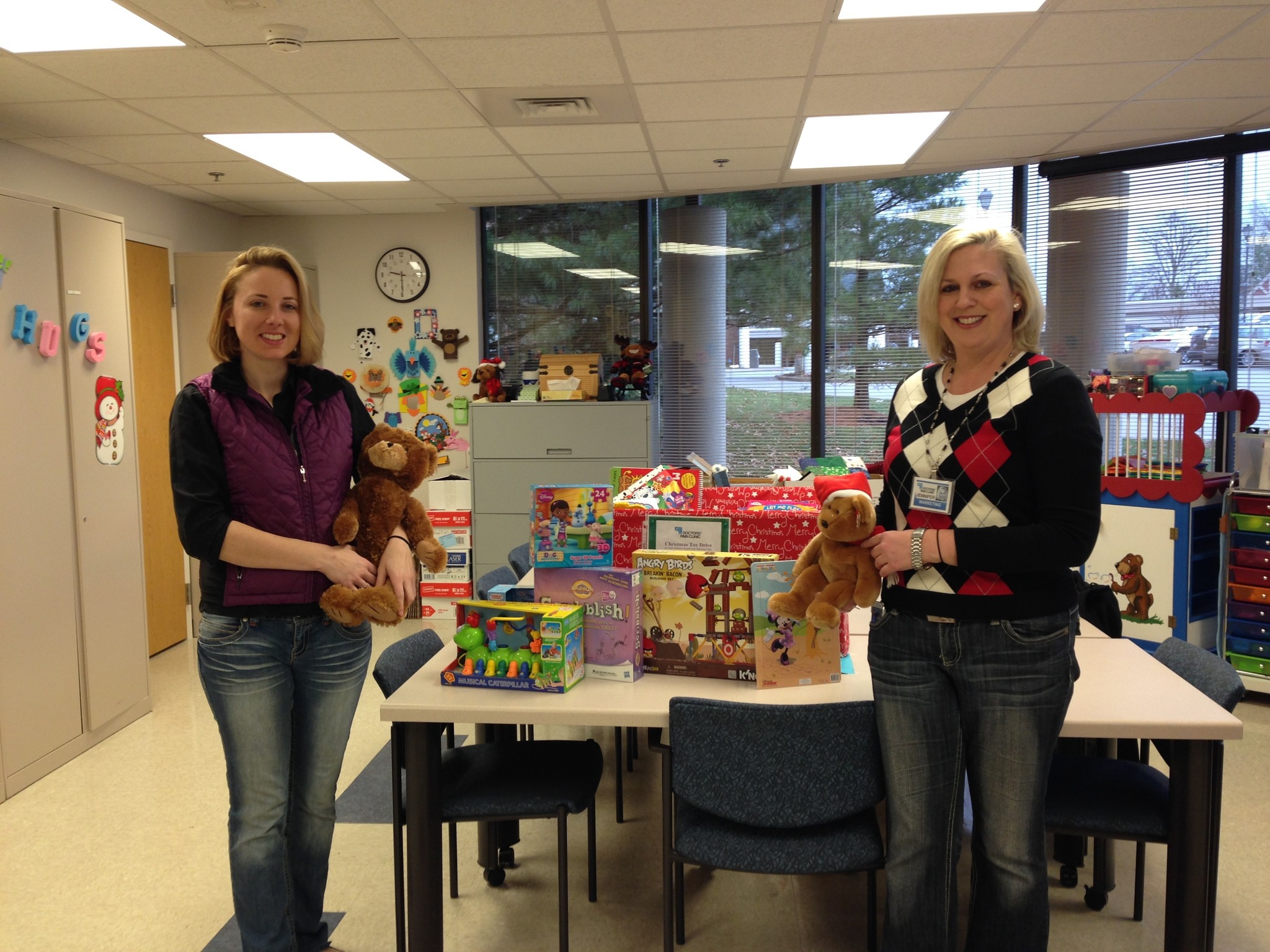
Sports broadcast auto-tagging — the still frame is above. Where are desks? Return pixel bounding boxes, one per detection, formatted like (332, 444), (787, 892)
(843, 586), (1112, 639)
(376, 627), (1244, 952)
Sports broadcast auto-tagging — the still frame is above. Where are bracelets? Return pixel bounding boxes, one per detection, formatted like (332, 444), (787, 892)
(911, 527), (933, 570)
(387, 535), (412, 552)
(937, 529), (944, 562)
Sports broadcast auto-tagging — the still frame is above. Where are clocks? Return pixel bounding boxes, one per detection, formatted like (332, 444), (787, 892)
(375, 247), (431, 303)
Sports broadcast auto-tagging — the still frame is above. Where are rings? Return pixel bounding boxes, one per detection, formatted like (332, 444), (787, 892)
(363, 582), (368, 588)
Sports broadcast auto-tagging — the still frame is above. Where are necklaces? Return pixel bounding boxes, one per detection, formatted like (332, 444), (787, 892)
(925, 345), (1018, 468)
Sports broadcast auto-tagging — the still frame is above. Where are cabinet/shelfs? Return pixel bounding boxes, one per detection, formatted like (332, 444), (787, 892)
(0, 186), (154, 808)
(470, 396), (659, 603)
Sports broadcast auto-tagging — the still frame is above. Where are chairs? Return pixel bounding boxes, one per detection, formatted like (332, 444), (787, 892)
(1044, 636), (1244, 952)
(645, 689), (885, 952)
(475, 565), (633, 827)
(371, 627), (602, 952)
(507, 542), (641, 776)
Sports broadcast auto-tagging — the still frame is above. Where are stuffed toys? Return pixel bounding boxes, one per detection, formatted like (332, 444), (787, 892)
(316, 420), (448, 628)
(610, 334), (658, 390)
(765, 490), (882, 630)
(470, 357), (507, 403)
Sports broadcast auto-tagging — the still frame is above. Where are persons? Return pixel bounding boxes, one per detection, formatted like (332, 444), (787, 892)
(167, 243), (420, 952)
(868, 220), (1106, 952)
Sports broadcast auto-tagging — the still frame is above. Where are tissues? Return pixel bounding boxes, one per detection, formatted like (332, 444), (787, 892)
(541, 376), (586, 401)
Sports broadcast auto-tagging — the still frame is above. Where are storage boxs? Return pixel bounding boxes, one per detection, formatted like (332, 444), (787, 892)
(1222, 494), (1270, 678)
(419, 549), (473, 583)
(419, 579), (473, 620)
(427, 472), (471, 510)
(525, 456), (884, 688)
(424, 509), (473, 551)
